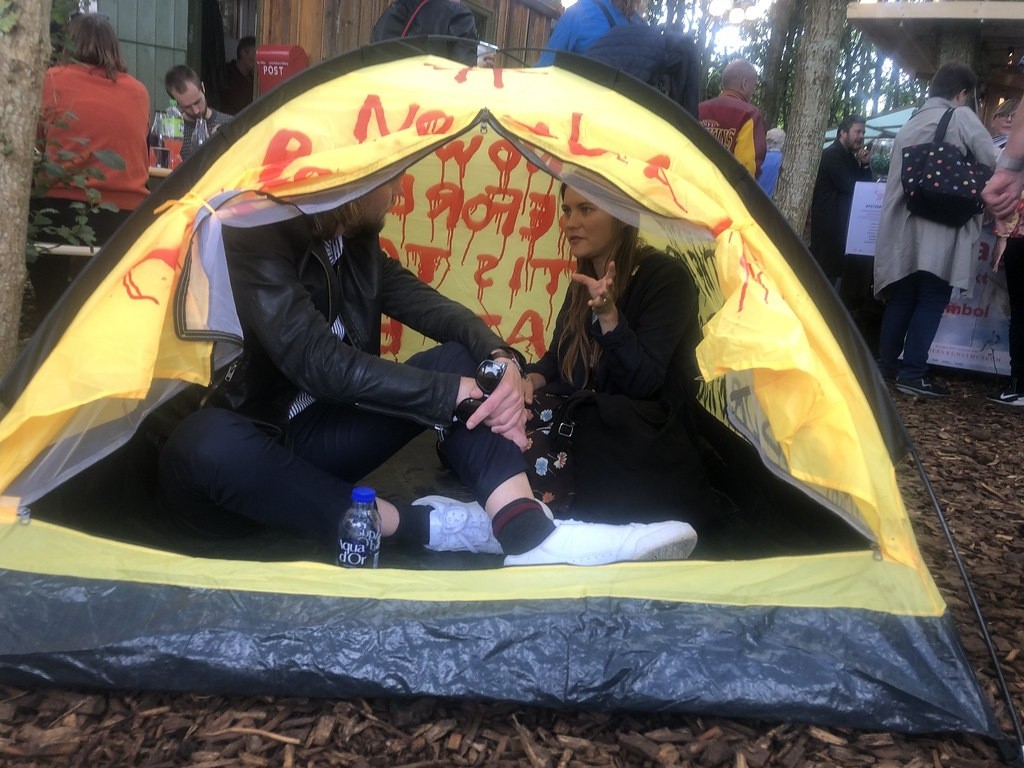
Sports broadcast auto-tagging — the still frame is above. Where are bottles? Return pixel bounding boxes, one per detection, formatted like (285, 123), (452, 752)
(162, 99), (182, 169)
(332, 486), (382, 569)
(191, 116), (210, 151)
(149, 110), (170, 147)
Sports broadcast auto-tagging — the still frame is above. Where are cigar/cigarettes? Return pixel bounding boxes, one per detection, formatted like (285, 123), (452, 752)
(863, 145), (868, 151)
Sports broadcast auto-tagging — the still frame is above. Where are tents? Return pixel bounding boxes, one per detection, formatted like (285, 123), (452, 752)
(0, 32), (1024, 764)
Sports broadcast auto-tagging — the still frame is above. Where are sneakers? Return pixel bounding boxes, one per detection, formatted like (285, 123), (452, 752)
(894, 375), (952, 397)
(503, 496), (698, 567)
(984, 388), (1024, 406)
(411, 494), (554, 553)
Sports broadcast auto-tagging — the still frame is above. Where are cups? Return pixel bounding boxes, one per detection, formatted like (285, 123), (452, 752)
(150, 148), (172, 169)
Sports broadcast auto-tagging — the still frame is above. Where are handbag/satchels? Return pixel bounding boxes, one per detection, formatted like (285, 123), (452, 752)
(901, 107), (989, 230)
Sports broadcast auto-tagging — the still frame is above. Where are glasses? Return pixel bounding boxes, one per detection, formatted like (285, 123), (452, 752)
(454, 359), (508, 430)
(997, 109), (1019, 122)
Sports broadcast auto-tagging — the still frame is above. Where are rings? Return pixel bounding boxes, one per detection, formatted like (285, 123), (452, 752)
(604, 295), (608, 302)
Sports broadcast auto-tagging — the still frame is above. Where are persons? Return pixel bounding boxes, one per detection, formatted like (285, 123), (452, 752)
(990, 97), (1024, 407)
(534, 0), (701, 124)
(125, 167), (700, 569)
(697, 58), (767, 182)
(436, 165), (705, 537)
(988, 96), (1020, 147)
(980, 99), (1024, 217)
(873, 60), (1003, 403)
(810, 114), (893, 362)
(213, 36), (256, 117)
(17, 11), (152, 324)
(369, 0), (478, 66)
(147, 65), (236, 170)
(757, 127), (786, 201)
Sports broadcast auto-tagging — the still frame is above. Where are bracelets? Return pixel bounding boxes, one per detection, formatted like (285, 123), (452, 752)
(995, 148), (1024, 172)
(490, 350), (527, 378)
